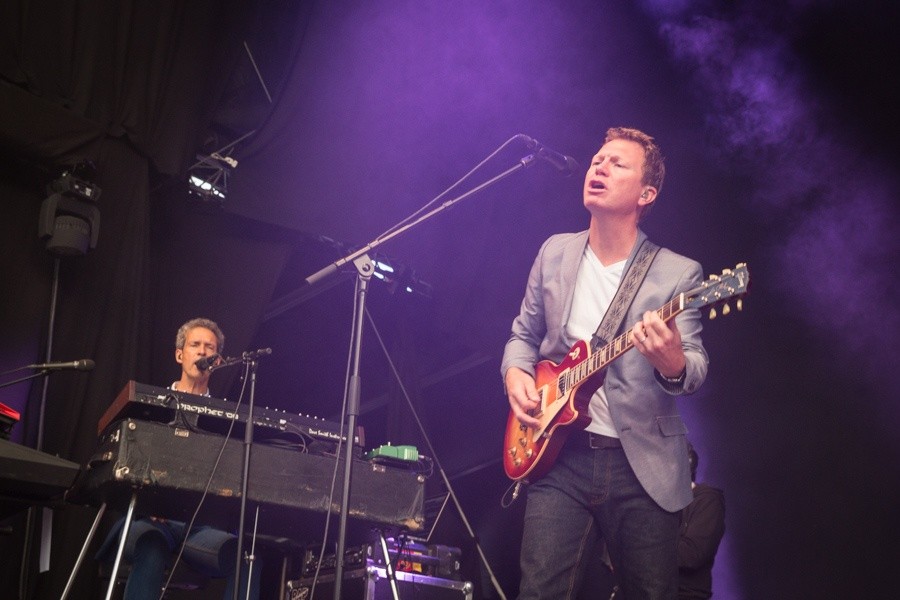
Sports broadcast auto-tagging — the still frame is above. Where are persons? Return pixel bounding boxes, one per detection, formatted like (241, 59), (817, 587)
(671, 440), (727, 600)
(107, 319), (265, 600)
(501, 128), (711, 600)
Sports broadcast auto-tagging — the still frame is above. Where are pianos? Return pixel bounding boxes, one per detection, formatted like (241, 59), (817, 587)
(98, 379), (367, 441)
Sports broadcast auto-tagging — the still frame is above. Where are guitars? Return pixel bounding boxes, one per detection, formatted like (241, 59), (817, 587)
(504, 261), (756, 484)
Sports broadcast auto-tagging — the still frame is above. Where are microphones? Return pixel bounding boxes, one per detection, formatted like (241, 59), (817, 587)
(520, 134), (580, 178)
(28, 359), (96, 371)
(195, 354), (220, 372)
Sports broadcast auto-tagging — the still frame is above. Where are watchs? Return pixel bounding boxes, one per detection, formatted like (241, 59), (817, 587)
(660, 368), (686, 384)
